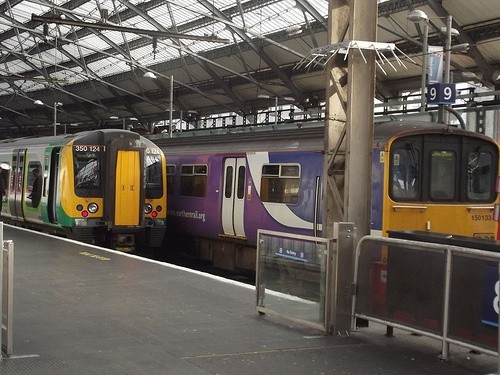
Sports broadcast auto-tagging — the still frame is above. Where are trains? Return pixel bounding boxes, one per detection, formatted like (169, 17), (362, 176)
(149, 118), (500, 349)
(0, 128), (168, 254)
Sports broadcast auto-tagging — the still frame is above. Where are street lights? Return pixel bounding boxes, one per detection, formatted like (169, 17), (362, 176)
(406, 10), (460, 114)
(109, 116), (139, 130)
(257, 94), (295, 125)
(52, 122), (78, 135)
(165, 108), (198, 133)
(143, 72), (174, 138)
(34, 99), (64, 136)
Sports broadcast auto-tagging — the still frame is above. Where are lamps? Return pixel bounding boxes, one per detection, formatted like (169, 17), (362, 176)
(406, 10), (428, 23)
(439, 26), (460, 37)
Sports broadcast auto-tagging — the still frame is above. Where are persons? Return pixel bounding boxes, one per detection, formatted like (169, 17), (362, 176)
(0, 167), (6, 212)
(29, 169), (42, 207)
